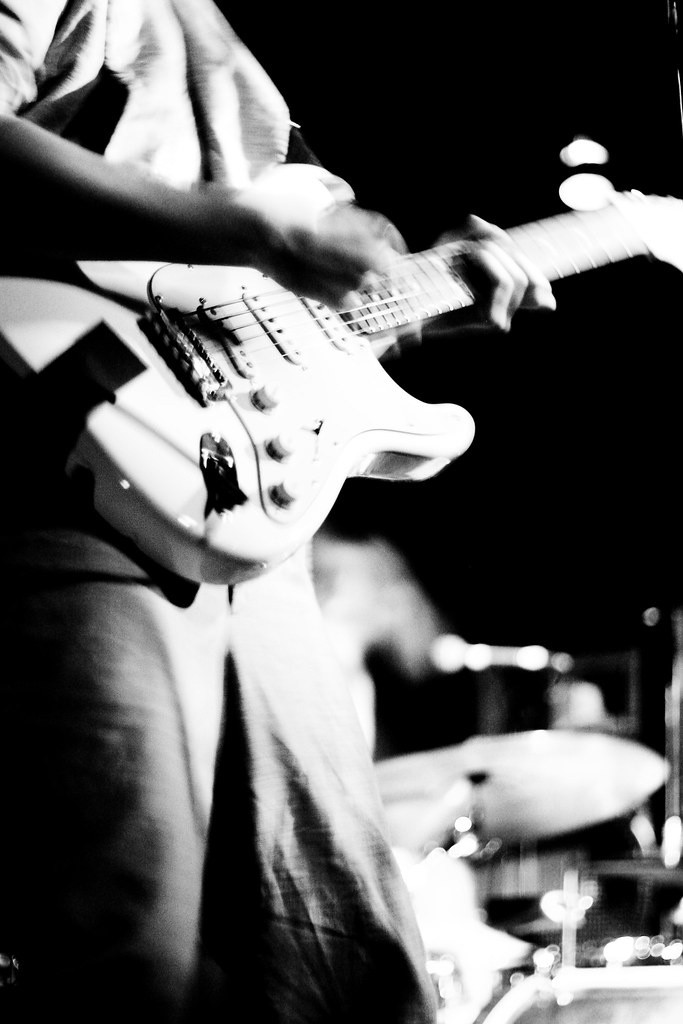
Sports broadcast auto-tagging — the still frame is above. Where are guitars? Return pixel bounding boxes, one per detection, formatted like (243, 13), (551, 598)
(0, 189), (682, 586)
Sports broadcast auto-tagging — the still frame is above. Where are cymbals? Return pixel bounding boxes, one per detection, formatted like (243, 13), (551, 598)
(358, 728), (671, 850)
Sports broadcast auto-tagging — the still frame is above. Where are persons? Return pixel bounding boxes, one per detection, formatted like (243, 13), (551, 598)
(0, 0), (556, 1024)
